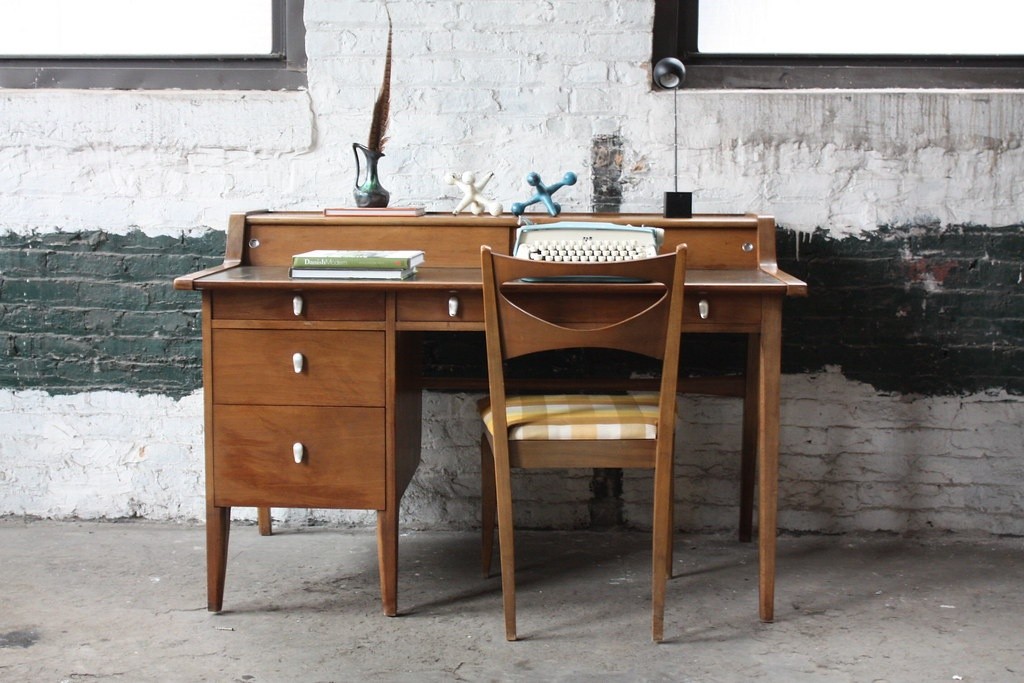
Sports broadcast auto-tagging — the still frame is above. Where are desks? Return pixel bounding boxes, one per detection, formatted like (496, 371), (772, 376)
(173, 209), (807, 623)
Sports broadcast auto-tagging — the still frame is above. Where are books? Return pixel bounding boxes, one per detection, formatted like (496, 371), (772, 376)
(288, 250), (426, 281)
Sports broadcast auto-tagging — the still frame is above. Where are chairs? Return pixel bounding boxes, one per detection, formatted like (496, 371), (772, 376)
(478, 245), (688, 641)
